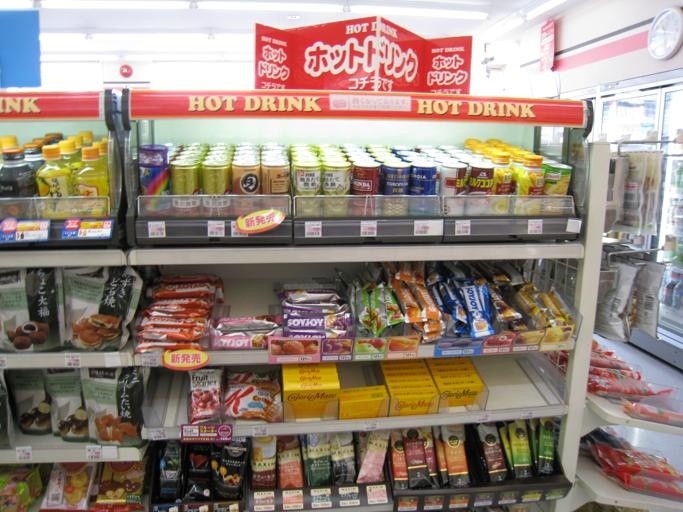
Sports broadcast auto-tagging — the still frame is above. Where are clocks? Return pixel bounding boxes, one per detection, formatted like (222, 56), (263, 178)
(648, 6), (683, 60)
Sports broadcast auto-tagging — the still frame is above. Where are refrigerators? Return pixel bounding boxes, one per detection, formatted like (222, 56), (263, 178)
(595, 76), (683, 375)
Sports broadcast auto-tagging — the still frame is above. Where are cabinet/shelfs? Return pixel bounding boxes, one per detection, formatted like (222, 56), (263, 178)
(122, 87), (612, 511)
(591, 127), (683, 512)
(2, 87), (154, 512)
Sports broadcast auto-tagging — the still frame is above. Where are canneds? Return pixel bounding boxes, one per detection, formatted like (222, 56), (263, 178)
(541, 163), (573, 216)
(137, 141), (495, 218)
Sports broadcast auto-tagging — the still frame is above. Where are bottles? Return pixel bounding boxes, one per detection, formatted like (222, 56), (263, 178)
(463, 138), (546, 217)
(661, 162), (683, 312)
(0, 131), (112, 220)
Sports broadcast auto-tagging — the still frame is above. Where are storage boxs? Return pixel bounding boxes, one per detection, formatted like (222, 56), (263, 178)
(281, 356), (485, 421)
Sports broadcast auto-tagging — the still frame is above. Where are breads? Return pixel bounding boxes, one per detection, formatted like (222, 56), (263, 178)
(7, 321), (49, 350)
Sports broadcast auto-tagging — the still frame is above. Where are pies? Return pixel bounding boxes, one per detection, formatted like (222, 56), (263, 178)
(74, 314), (120, 349)
